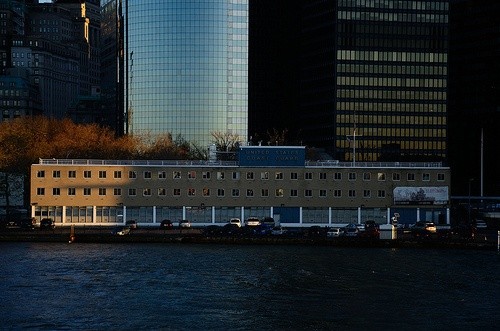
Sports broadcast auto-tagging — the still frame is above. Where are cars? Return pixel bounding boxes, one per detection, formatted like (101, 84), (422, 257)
(343, 223), (365, 237)
(160, 219), (174, 230)
(178, 220), (191, 228)
(326, 227), (344, 237)
(39, 218), (55, 229)
(470, 219), (487, 228)
(125, 220), (138, 229)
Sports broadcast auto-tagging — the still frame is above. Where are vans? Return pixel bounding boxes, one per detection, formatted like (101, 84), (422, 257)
(228, 218), (241, 227)
(411, 222), (438, 232)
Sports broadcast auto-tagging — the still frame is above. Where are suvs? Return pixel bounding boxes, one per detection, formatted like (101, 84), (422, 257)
(245, 216), (261, 226)
(259, 217), (276, 226)
